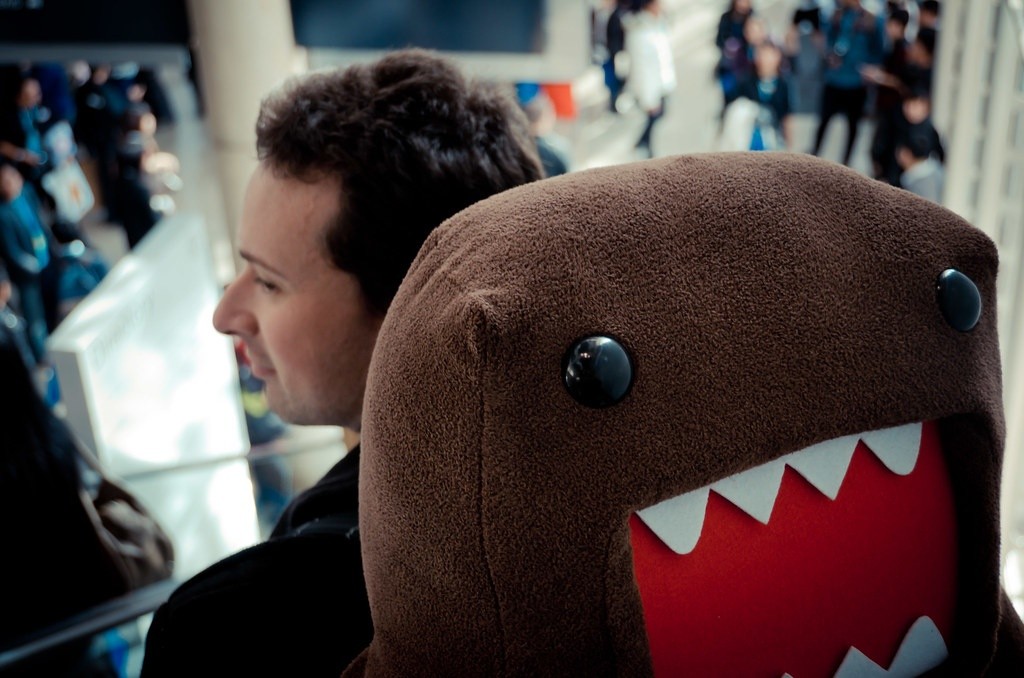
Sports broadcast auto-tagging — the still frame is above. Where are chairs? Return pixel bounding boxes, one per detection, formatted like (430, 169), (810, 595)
(350, 151), (1024, 678)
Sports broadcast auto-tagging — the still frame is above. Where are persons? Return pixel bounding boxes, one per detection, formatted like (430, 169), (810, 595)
(711, 1), (945, 204)
(604, 0), (674, 157)
(1, 52), (179, 497)
(137, 48), (546, 678)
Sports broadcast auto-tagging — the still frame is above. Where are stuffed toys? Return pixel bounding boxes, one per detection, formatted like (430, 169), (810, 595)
(354, 149), (1024, 678)
